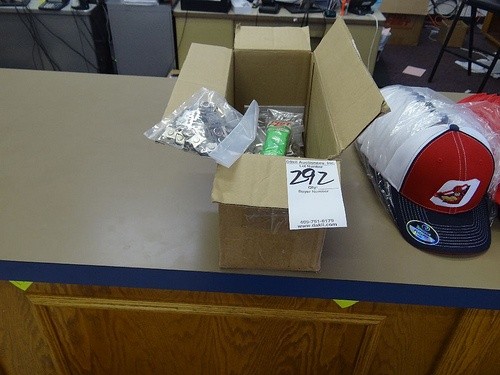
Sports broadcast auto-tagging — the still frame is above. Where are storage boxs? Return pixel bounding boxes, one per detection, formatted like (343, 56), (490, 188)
(437, 20), (468, 48)
(379, 0), (429, 48)
(154, 18), (391, 271)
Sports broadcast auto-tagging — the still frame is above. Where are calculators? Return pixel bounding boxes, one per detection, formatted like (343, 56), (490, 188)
(39, 0), (69, 11)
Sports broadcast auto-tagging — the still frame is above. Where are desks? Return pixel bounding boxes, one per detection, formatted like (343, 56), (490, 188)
(173, 0), (387, 77)
(0, 0), (100, 74)
(0, 68), (500, 374)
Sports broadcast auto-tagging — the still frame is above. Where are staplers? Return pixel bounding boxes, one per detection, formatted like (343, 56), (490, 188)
(252, 0), (260, 8)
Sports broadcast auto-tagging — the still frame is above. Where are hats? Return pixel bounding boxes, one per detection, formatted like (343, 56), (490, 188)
(356, 84), (500, 230)
(384, 123), (496, 258)
